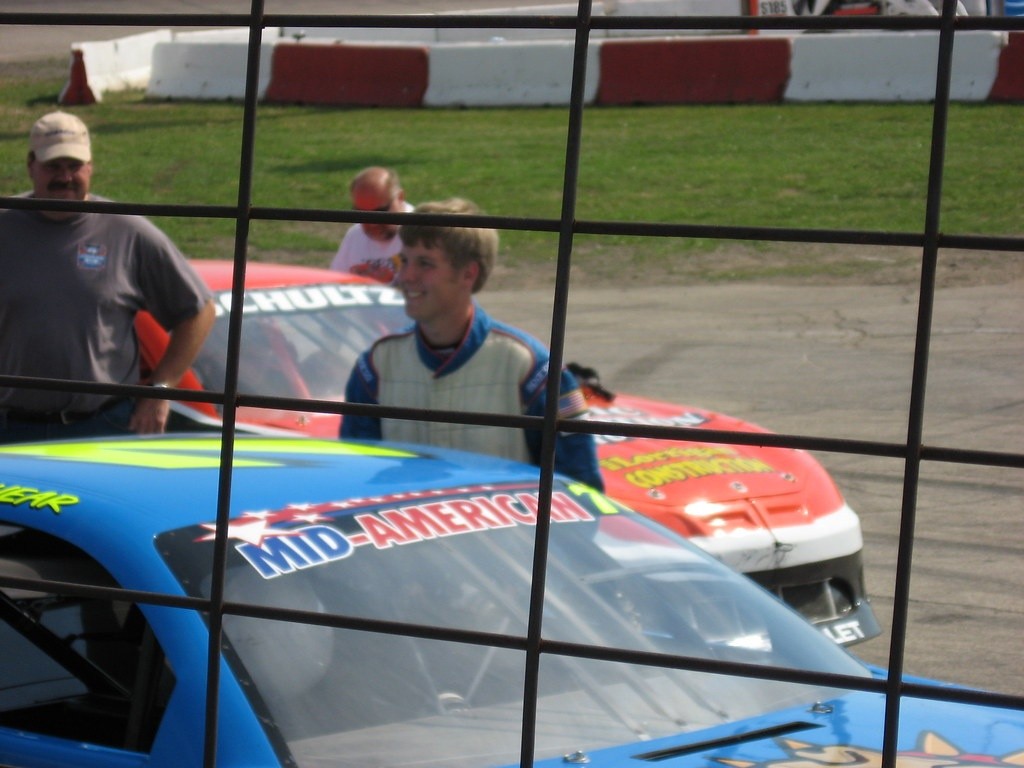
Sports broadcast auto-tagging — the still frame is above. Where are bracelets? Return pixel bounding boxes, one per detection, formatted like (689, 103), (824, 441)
(149, 383), (170, 387)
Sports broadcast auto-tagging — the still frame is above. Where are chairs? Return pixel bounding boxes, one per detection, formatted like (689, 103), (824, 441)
(198, 566), (343, 740)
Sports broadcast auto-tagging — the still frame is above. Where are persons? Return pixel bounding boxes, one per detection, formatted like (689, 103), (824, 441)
(329, 167), (417, 281)
(0, 108), (215, 441)
(338, 196), (605, 541)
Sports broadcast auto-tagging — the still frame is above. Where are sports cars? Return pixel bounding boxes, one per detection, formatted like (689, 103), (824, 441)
(1, 435), (1023, 767)
(132, 260), (888, 653)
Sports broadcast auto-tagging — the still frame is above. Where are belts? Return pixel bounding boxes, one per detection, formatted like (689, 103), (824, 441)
(0, 394), (126, 426)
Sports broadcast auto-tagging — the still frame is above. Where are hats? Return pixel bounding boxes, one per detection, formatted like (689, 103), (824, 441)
(26, 112), (91, 163)
